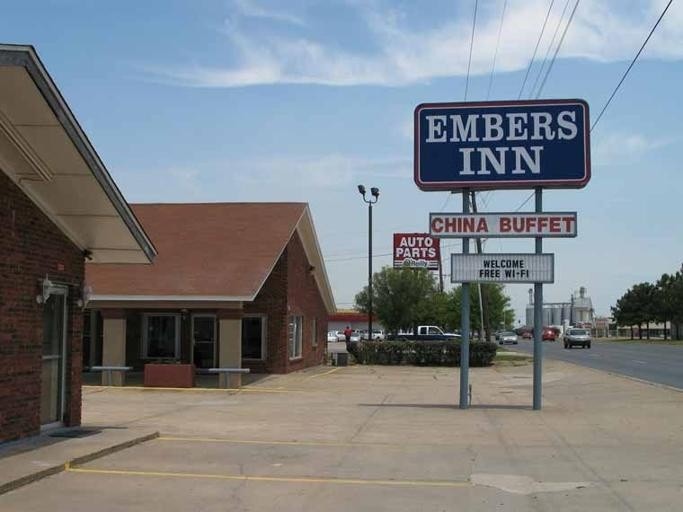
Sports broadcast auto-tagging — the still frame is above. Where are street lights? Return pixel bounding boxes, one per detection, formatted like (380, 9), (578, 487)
(356, 185), (378, 332)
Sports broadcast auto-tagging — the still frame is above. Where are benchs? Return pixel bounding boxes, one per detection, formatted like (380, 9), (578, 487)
(87, 365), (133, 385)
(209, 366), (250, 389)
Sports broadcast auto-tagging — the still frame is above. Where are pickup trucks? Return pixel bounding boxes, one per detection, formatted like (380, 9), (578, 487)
(387, 325), (462, 337)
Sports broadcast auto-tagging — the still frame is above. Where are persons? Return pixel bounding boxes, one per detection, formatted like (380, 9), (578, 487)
(344, 326), (352, 343)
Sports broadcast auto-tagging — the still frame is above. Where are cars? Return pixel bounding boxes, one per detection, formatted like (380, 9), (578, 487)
(562, 328), (591, 348)
(326, 328), (386, 342)
(493, 326), (555, 345)
